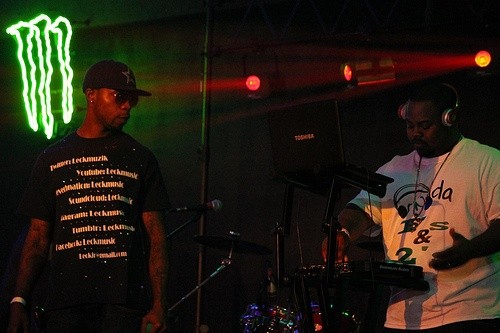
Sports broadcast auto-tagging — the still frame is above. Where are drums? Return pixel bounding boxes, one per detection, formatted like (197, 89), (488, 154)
(296, 303), (359, 333)
(242, 302), (300, 333)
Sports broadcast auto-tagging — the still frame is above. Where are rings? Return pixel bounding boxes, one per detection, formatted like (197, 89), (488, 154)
(448, 263), (455, 268)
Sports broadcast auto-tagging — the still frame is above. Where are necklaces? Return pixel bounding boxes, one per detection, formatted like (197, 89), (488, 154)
(412, 137), (452, 216)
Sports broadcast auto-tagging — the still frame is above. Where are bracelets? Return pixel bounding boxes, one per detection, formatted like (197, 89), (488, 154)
(338, 228), (350, 237)
(9, 296), (26, 304)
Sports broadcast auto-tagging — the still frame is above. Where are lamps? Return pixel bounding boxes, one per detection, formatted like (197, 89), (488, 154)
(242, 47), (278, 99)
(339, 57), (396, 90)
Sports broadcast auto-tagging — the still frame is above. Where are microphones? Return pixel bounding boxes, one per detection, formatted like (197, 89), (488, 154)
(168, 199), (224, 213)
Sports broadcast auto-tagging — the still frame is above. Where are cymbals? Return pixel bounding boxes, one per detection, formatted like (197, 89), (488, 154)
(192, 235), (273, 256)
(357, 241), (384, 252)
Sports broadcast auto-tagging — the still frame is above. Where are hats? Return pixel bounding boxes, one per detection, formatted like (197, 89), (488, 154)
(82, 60), (152, 96)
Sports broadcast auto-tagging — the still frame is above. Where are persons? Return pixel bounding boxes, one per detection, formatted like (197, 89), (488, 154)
(320, 78), (500, 333)
(7, 59), (175, 333)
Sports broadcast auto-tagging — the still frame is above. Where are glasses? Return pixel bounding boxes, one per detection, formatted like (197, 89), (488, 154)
(112, 91), (139, 106)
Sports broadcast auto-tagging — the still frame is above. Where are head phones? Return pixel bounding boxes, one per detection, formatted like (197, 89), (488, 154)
(397, 77), (463, 128)
